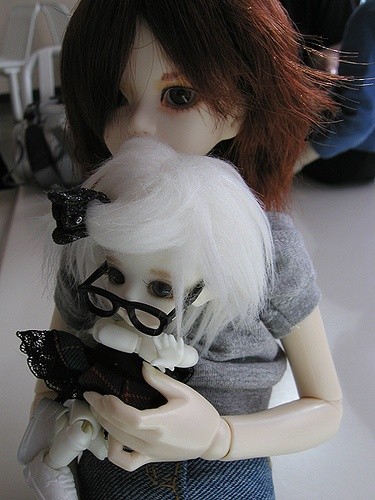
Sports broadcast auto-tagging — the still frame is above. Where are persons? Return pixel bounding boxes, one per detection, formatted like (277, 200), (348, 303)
(15, 137), (277, 499)
(23, 0), (375, 499)
(292, 0), (375, 186)
